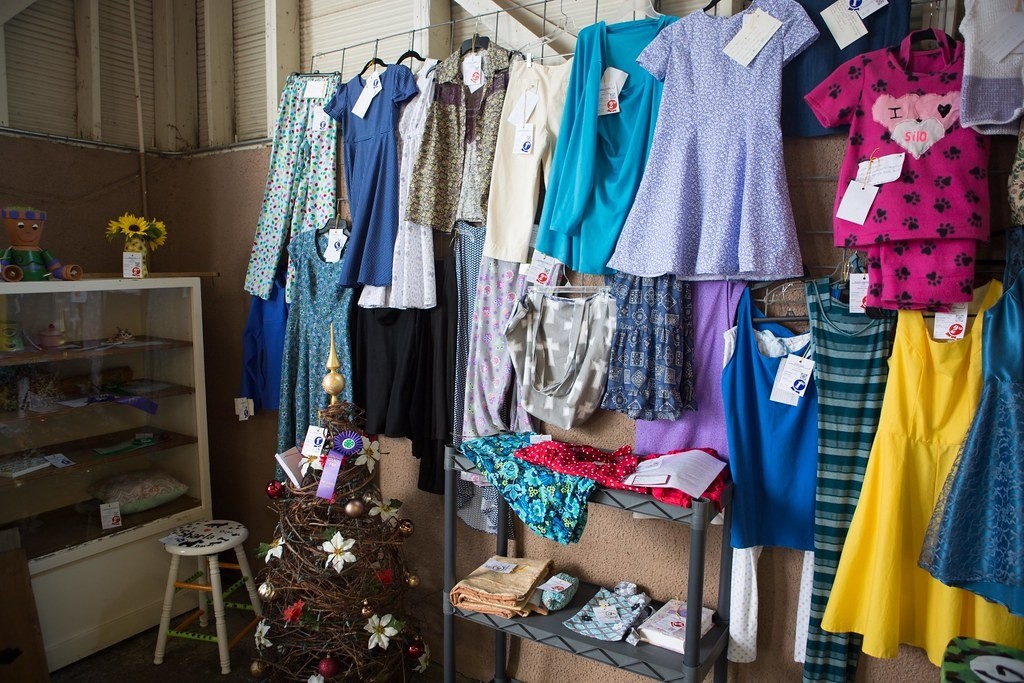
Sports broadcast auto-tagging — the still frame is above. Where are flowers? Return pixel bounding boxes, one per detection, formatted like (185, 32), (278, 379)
(257, 536), (285, 562)
(322, 491), (337, 514)
(320, 454), (348, 467)
(321, 528), (356, 574)
(366, 567), (395, 595)
(355, 436), (382, 473)
(363, 614), (405, 650)
(107, 212), (167, 279)
(297, 449), (323, 477)
(369, 497), (402, 522)
(254, 618), (334, 656)
(284, 600), (345, 631)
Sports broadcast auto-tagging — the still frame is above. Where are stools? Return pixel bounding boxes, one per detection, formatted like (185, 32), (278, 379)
(154, 520), (262, 674)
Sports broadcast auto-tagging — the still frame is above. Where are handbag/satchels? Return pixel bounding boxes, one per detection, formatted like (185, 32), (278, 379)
(502, 290), (618, 430)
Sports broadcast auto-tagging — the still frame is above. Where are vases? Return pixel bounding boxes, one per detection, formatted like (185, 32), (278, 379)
(123, 235), (148, 279)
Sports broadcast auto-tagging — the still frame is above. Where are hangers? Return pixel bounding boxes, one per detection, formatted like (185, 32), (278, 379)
(604, 0), (665, 25)
(290, 52), (342, 78)
(528, 263), (613, 303)
(396, 31), (441, 64)
(829, 249), (858, 285)
(756, 284), (809, 322)
(889, 0), (957, 53)
(358, 41), (388, 74)
(426, 14), (512, 78)
(511, 0), (583, 64)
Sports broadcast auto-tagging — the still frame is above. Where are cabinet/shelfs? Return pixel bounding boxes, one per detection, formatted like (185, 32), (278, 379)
(0, 277), (213, 683)
(443, 443), (732, 683)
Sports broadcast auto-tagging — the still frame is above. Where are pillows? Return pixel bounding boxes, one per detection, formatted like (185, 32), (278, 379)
(86, 469), (191, 515)
